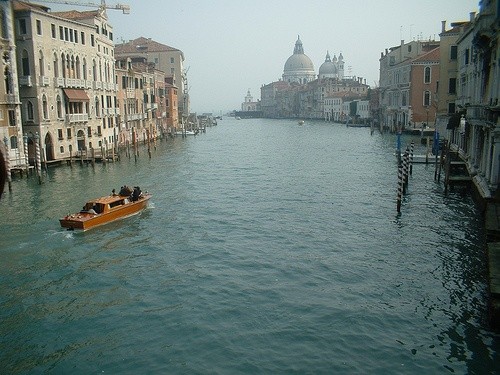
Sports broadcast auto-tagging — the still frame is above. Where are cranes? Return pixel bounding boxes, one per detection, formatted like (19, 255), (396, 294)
(27, 0), (130, 16)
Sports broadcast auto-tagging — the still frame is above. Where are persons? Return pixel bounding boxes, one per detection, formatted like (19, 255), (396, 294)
(83, 203), (97, 214)
(112, 185), (141, 202)
(420, 122), (426, 128)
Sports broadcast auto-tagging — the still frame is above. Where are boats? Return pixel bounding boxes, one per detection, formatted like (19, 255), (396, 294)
(59, 188), (151, 232)
(173, 115), (222, 137)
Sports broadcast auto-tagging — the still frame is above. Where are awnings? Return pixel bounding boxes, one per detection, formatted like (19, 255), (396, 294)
(63, 88), (90, 102)
